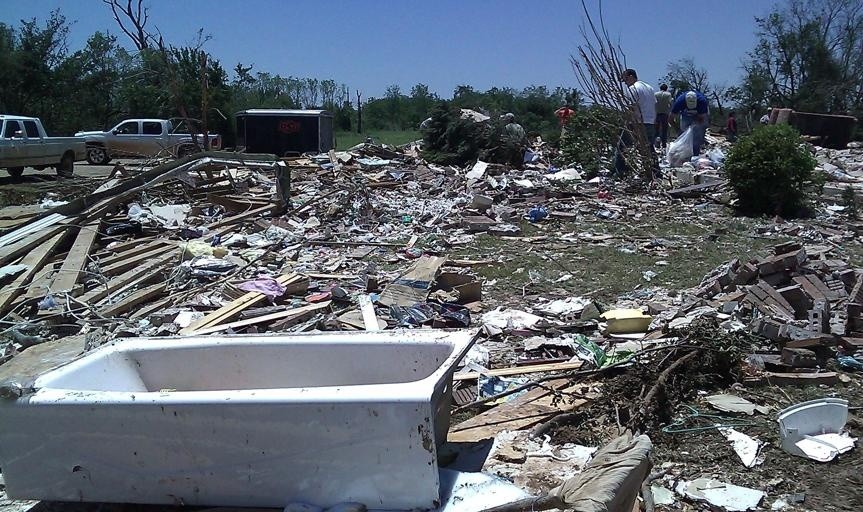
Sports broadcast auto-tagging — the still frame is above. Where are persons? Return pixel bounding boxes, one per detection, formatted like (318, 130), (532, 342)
(668, 88), (713, 132)
(726, 111), (737, 139)
(654, 83), (673, 149)
(553, 105), (575, 139)
(611, 69), (658, 181)
(499, 113), (527, 148)
(759, 107), (772, 123)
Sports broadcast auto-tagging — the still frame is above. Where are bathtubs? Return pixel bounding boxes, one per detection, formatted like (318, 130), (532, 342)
(0, 327), (482, 512)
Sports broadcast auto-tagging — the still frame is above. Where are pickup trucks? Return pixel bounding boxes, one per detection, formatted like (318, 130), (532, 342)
(0, 114), (86, 176)
(74, 119), (221, 166)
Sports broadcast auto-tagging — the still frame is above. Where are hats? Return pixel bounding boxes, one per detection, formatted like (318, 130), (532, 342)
(685, 91), (697, 109)
(500, 112), (515, 119)
